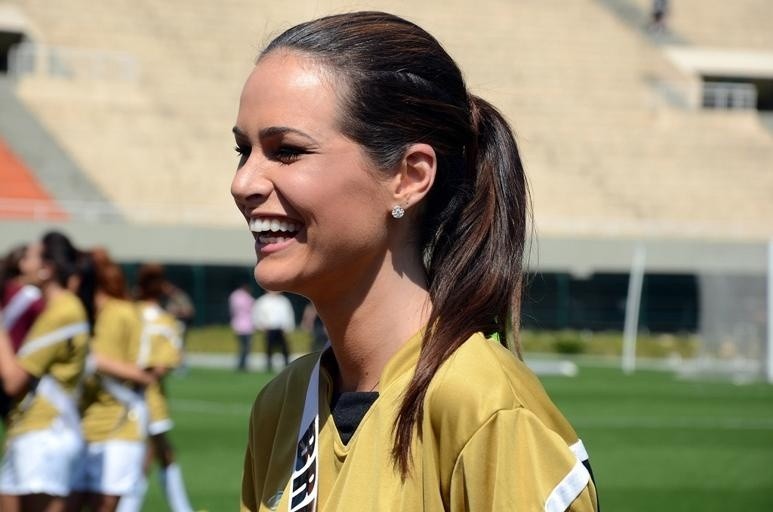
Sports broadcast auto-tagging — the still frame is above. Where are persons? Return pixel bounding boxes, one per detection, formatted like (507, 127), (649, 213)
(301, 302), (328, 353)
(228, 9), (597, 510)
(227, 281), (254, 369)
(250, 291), (296, 372)
